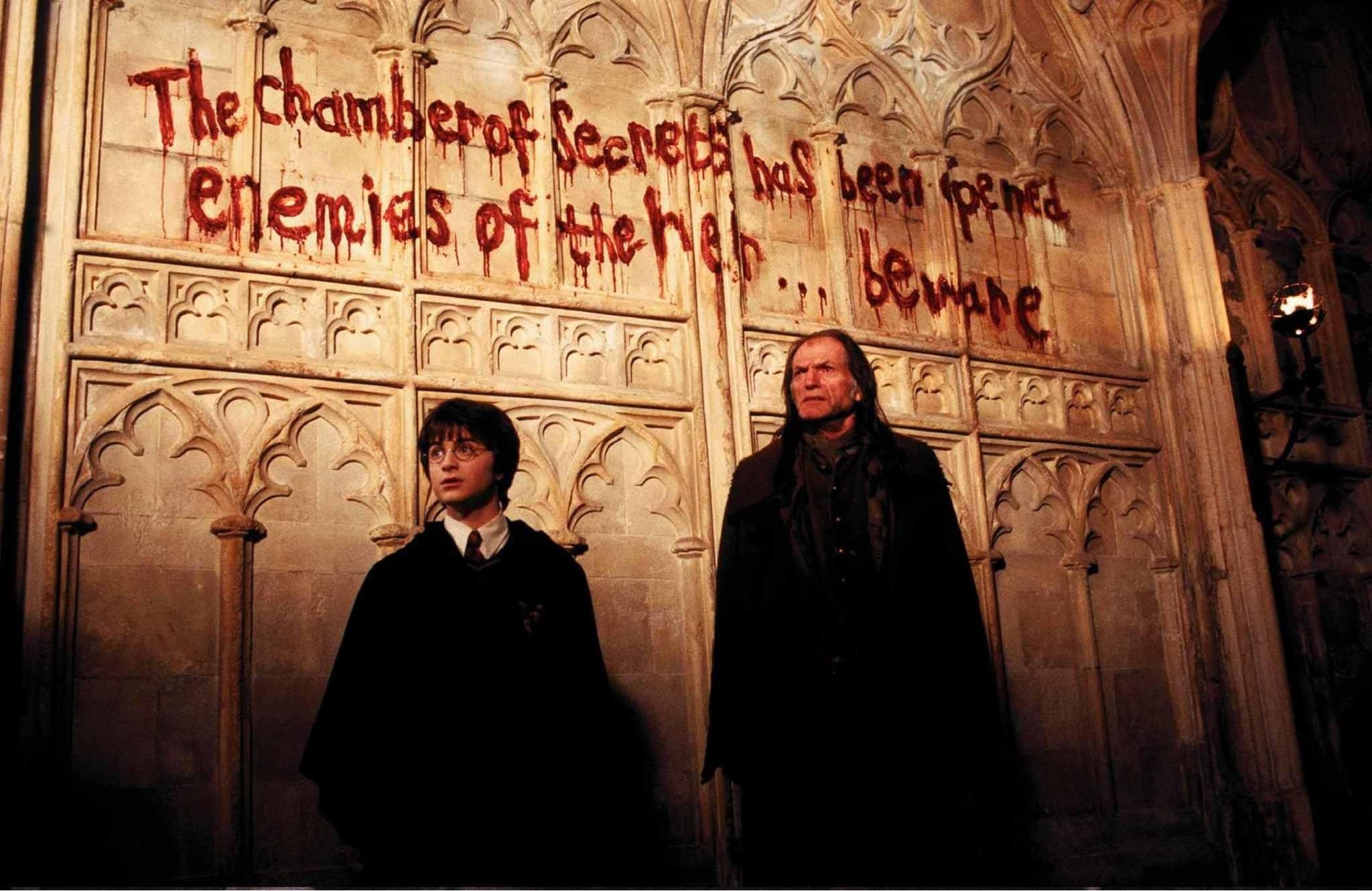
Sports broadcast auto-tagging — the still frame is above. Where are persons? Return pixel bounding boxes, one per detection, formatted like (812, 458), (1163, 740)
(699, 328), (1018, 891)
(299, 397), (613, 890)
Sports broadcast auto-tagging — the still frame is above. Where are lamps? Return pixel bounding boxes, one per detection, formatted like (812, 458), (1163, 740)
(1226, 281), (1327, 522)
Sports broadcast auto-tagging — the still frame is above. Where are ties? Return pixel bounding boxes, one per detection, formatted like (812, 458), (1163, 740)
(464, 530), (487, 567)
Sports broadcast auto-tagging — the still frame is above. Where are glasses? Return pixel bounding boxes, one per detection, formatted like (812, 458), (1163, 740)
(423, 442), (490, 464)
